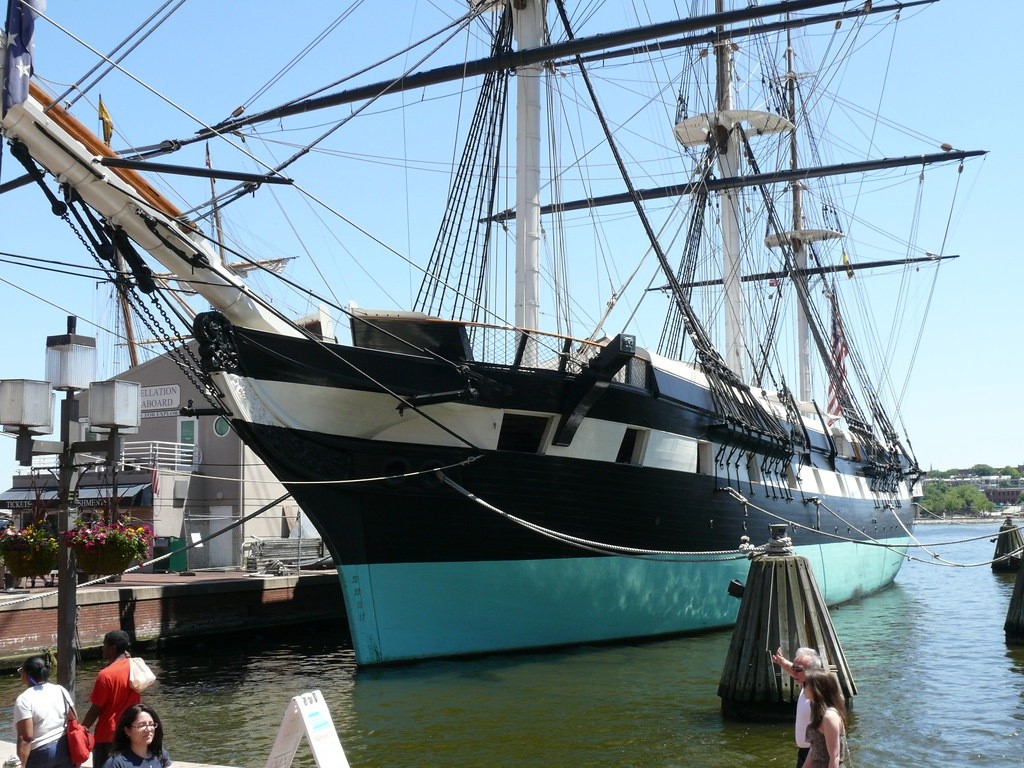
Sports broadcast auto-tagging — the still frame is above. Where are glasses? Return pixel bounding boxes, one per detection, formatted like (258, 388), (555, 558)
(18, 668), (26, 673)
(791, 665), (808, 673)
(129, 723), (158, 732)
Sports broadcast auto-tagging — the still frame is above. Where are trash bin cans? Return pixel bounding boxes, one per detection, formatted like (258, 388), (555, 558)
(153, 535), (187, 572)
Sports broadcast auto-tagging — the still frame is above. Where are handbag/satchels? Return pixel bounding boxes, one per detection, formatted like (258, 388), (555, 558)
(129, 657), (157, 694)
(66, 717), (95, 766)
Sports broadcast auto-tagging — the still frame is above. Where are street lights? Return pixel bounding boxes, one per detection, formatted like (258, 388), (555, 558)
(0, 313), (144, 707)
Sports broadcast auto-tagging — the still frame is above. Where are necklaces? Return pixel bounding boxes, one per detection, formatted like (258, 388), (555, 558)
(135, 749), (153, 768)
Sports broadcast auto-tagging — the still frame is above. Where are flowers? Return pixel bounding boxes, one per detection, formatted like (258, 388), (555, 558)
(66, 512), (157, 569)
(0, 525), (59, 566)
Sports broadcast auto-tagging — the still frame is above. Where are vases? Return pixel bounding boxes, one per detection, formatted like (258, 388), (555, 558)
(74, 544), (137, 576)
(3, 550), (58, 576)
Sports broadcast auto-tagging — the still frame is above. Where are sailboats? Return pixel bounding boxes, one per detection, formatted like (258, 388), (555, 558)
(0, 1), (992, 673)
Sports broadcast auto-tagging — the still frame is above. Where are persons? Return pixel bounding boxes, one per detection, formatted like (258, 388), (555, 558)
(81, 630), (174, 768)
(0, 520), (29, 539)
(13, 656), (79, 768)
(772, 647), (855, 768)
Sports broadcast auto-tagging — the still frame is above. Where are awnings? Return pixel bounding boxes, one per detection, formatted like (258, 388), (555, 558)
(0, 483), (153, 508)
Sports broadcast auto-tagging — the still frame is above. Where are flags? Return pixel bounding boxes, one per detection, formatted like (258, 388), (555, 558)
(825, 283), (849, 428)
(99, 101), (113, 148)
